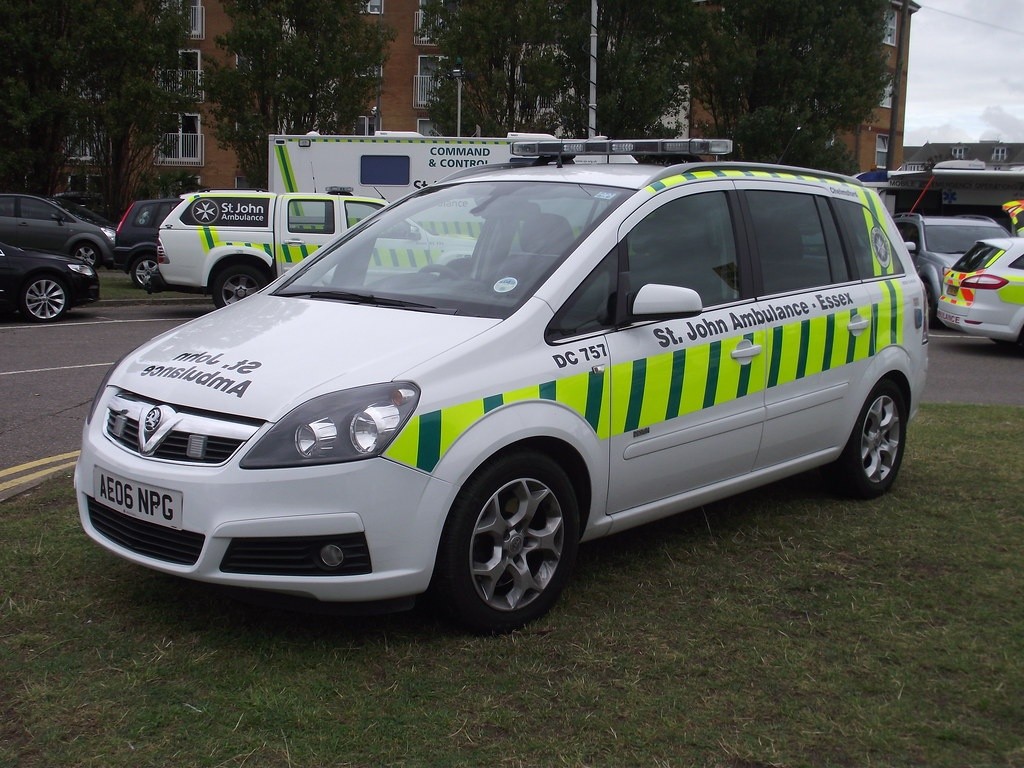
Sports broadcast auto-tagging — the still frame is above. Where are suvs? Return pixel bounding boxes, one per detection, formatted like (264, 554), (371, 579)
(111, 197), (186, 291)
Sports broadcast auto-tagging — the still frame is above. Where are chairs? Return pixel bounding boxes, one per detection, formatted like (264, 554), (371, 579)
(489, 213), (574, 299)
(745, 214), (828, 295)
(327, 206), (348, 234)
(629, 203), (740, 309)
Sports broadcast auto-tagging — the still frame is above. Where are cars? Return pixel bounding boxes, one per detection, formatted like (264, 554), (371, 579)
(0, 191), (118, 272)
(891, 211), (1015, 331)
(0, 242), (102, 324)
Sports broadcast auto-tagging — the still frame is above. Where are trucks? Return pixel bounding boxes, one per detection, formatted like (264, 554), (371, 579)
(265, 128), (701, 288)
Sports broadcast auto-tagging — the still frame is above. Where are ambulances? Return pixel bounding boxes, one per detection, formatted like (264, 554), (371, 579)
(935, 200), (1024, 353)
(71, 134), (932, 639)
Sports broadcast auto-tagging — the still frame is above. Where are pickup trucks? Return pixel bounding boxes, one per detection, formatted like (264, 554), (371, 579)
(152, 185), (476, 310)
